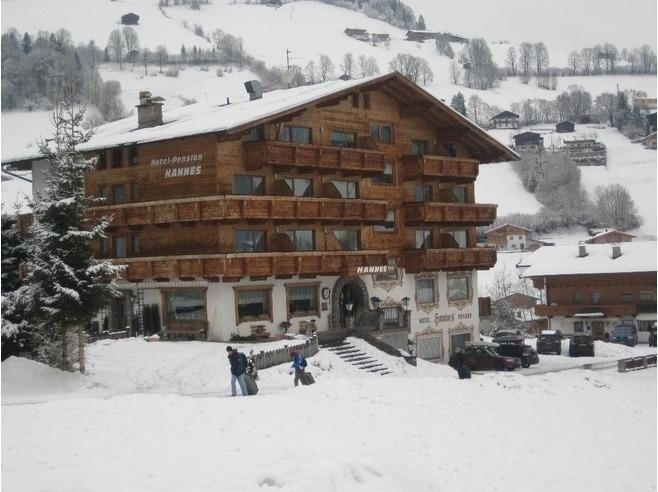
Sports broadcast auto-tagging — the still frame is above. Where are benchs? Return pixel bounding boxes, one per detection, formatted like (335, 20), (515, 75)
(158, 319), (208, 340)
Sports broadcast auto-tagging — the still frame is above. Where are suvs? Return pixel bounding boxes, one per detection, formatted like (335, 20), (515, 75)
(448, 347), (523, 371)
(610, 324), (638, 346)
(568, 335), (594, 356)
(648, 322), (657, 347)
(494, 344), (539, 368)
(537, 330), (561, 355)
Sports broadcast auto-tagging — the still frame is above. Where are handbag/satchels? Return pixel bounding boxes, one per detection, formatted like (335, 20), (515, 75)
(303, 360), (307, 366)
(241, 352), (247, 368)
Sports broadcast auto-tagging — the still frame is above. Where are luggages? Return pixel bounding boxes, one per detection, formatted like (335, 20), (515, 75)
(244, 374), (258, 395)
(300, 372), (315, 385)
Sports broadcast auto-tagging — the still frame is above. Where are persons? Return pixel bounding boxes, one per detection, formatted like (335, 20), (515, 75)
(647, 323), (657, 334)
(226, 346), (247, 396)
(290, 350), (307, 386)
(454, 346), (468, 371)
